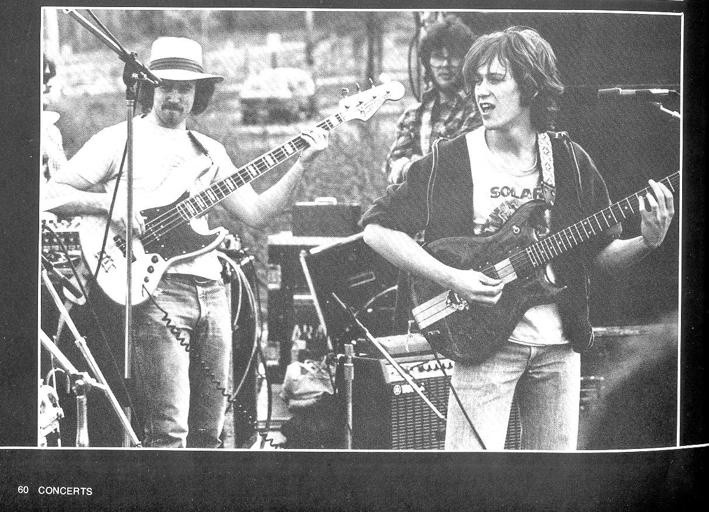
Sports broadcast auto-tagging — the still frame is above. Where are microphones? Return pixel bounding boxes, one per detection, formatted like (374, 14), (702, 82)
(596, 87), (677, 100)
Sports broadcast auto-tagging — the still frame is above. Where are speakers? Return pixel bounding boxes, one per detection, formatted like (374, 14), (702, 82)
(263, 200), (676, 451)
(41, 276), (125, 447)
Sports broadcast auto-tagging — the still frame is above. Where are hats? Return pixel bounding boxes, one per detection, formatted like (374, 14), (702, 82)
(146, 36), (225, 83)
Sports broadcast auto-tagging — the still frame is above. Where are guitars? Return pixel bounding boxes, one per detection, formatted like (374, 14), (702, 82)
(80, 78), (406, 306)
(409, 172), (679, 364)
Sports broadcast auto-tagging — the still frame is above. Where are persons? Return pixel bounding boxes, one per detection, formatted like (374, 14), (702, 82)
(41, 36), (331, 448)
(379, 14), (483, 338)
(355, 23), (676, 450)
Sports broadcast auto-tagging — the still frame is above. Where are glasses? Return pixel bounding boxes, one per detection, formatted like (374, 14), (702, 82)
(429, 56), (461, 67)
